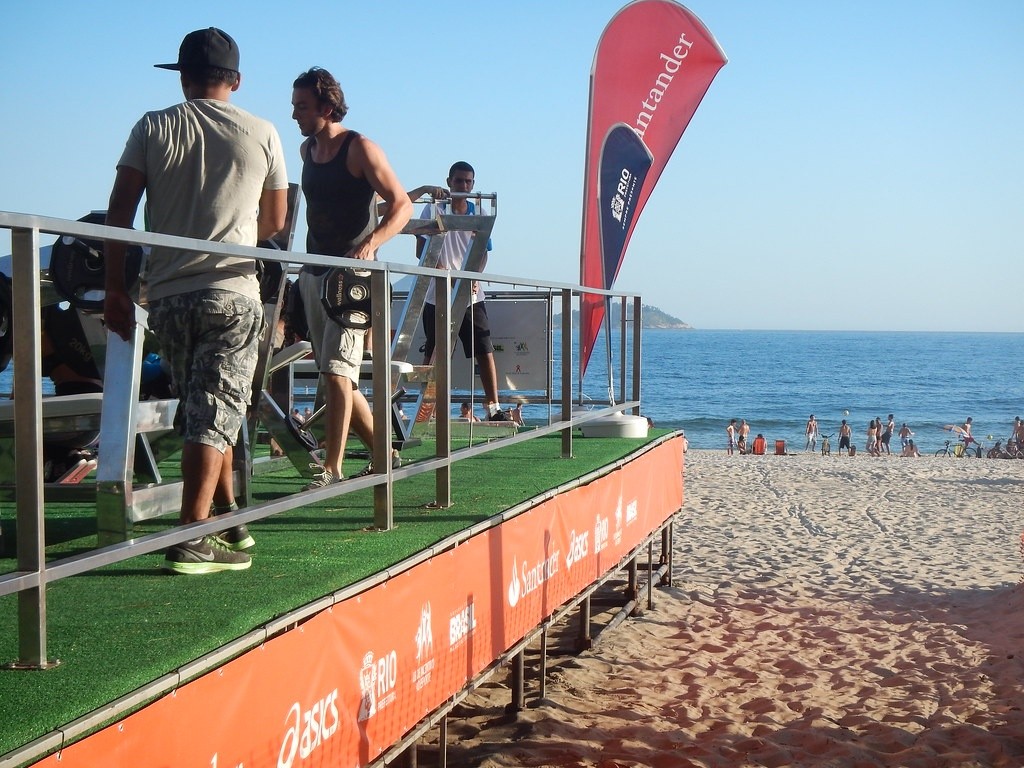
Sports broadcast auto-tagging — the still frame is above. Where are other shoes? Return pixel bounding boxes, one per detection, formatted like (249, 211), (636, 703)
(488, 410), (509, 421)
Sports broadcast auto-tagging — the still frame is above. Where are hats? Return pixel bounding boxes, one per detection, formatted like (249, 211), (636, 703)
(154, 26), (240, 72)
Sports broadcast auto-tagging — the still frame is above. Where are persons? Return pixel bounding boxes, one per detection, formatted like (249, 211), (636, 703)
(0, 161), (526, 493)
(641, 414), (1024, 459)
(101, 28), (286, 576)
(289, 67), (414, 496)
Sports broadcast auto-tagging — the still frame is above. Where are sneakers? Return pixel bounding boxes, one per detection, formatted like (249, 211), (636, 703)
(349, 449), (402, 479)
(44, 449), (97, 483)
(301, 463), (345, 492)
(208, 507), (255, 551)
(165, 536), (256, 576)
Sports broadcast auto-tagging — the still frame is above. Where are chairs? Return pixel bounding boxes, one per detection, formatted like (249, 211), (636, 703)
(753, 438), (766, 455)
(774, 440), (787, 455)
